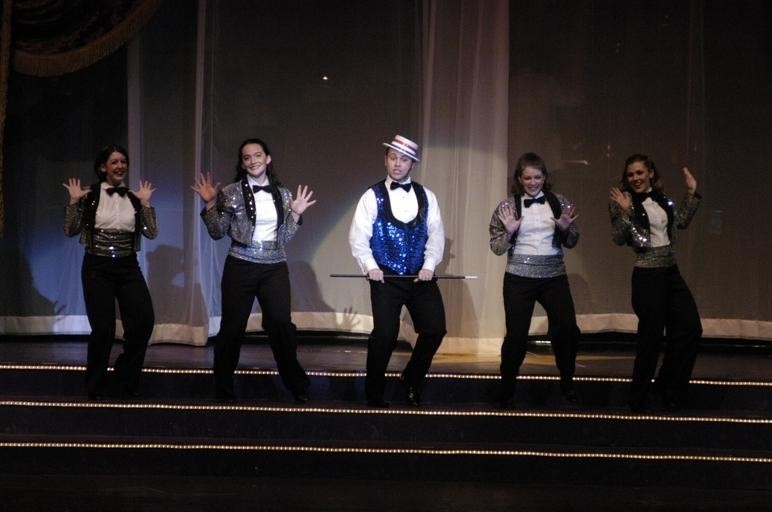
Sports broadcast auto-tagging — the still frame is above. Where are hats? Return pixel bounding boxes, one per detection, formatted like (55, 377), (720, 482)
(381, 134), (420, 165)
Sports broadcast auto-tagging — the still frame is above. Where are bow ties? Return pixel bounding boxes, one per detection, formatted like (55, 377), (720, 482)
(107, 188), (129, 197)
(391, 182), (411, 192)
(638, 192), (656, 201)
(253, 185), (272, 193)
(524, 196), (545, 207)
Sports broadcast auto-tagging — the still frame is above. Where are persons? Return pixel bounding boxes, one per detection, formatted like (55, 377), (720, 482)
(487, 152), (580, 408)
(62, 143), (159, 400)
(605, 152), (704, 415)
(346, 134), (448, 409)
(189, 137), (317, 404)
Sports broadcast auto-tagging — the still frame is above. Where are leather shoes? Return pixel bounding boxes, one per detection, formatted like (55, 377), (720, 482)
(366, 397), (390, 407)
(125, 381), (142, 398)
(561, 379), (577, 403)
(400, 374), (420, 407)
(293, 388), (309, 402)
(498, 388), (514, 409)
(88, 390), (102, 401)
(630, 390), (681, 412)
